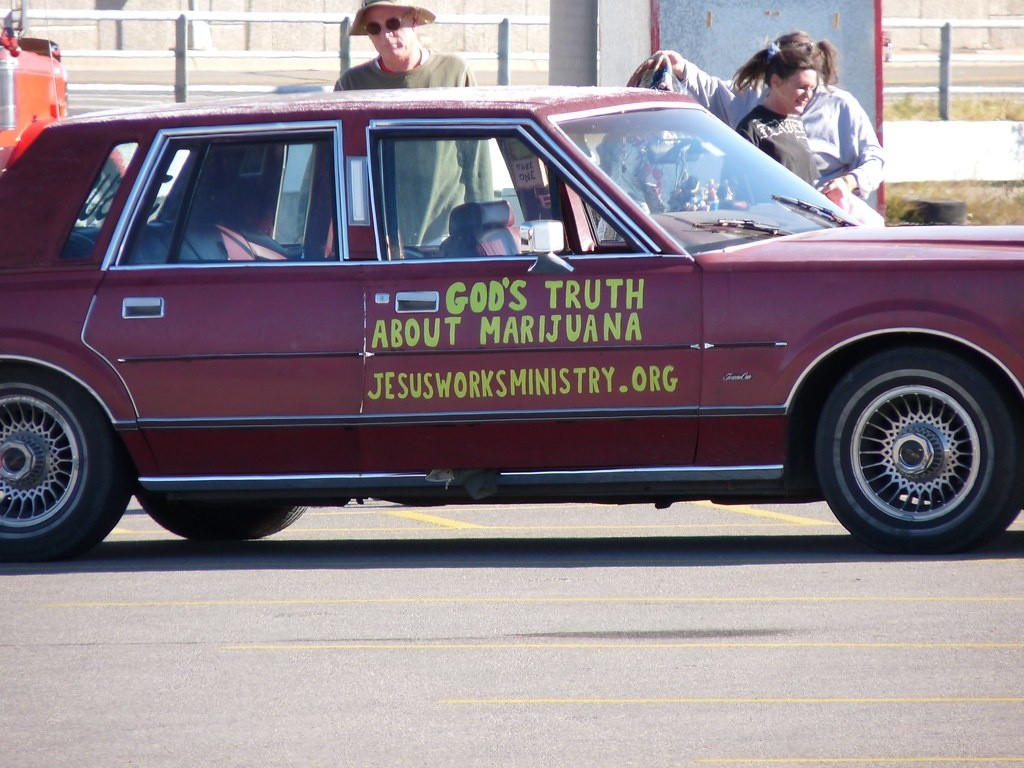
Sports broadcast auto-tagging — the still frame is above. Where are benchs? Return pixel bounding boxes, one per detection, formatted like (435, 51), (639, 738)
(65, 213), (288, 265)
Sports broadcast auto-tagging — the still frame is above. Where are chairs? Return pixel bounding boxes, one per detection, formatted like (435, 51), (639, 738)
(440, 202), (517, 256)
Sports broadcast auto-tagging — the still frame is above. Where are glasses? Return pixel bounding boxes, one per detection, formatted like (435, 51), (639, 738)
(364, 12), (413, 35)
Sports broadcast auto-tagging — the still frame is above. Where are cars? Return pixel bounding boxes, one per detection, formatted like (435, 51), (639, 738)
(0, 85), (1024, 567)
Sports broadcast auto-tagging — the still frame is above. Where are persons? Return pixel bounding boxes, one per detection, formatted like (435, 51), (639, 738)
(330, 0), (495, 249)
(718, 47), (819, 207)
(637, 30), (891, 202)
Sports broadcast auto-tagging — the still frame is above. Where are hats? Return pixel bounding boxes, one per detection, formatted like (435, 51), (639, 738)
(349, 0), (436, 35)
